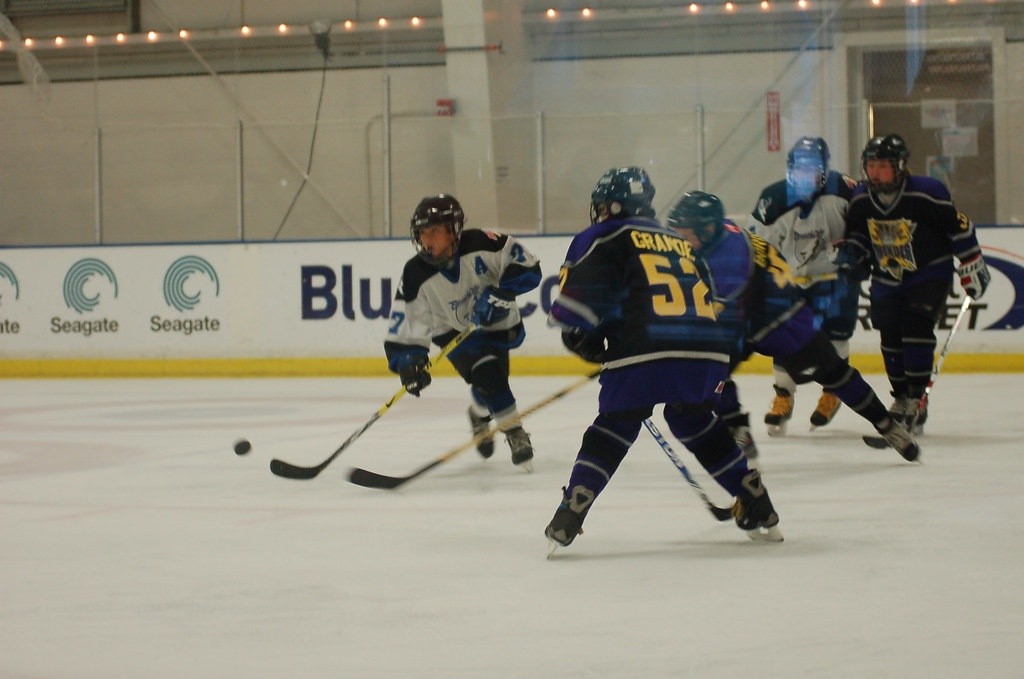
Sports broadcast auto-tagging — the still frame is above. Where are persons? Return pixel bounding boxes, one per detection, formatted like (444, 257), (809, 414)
(668, 190), (919, 471)
(542, 166), (783, 548)
(386, 193), (541, 469)
(751, 135), (859, 434)
(840, 133), (992, 434)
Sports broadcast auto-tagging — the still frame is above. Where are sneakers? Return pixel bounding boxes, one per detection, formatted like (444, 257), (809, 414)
(890, 391), (906, 422)
(765, 385), (794, 437)
(469, 404), (494, 458)
(876, 416), (923, 462)
(544, 486), (595, 558)
(504, 425), (534, 473)
(904, 396), (928, 435)
(809, 391), (841, 431)
(735, 469), (784, 543)
(730, 413), (757, 460)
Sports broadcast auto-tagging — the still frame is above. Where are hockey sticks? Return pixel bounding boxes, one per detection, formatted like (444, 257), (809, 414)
(345, 367), (603, 490)
(643, 418), (741, 523)
(269, 315), (480, 480)
(861, 287), (977, 451)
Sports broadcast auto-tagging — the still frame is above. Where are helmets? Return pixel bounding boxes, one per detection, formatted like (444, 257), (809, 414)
(667, 190), (723, 250)
(589, 166), (655, 226)
(860, 134), (907, 193)
(410, 193), (465, 265)
(787, 136), (830, 201)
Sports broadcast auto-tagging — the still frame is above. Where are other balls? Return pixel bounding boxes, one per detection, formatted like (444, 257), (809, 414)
(234, 440), (251, 455)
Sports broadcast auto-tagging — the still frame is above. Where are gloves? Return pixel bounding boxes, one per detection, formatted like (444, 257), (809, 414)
(399, 355), (431, 397)
(958, 254), (990, 300)
(476, 285), (516, 326)
